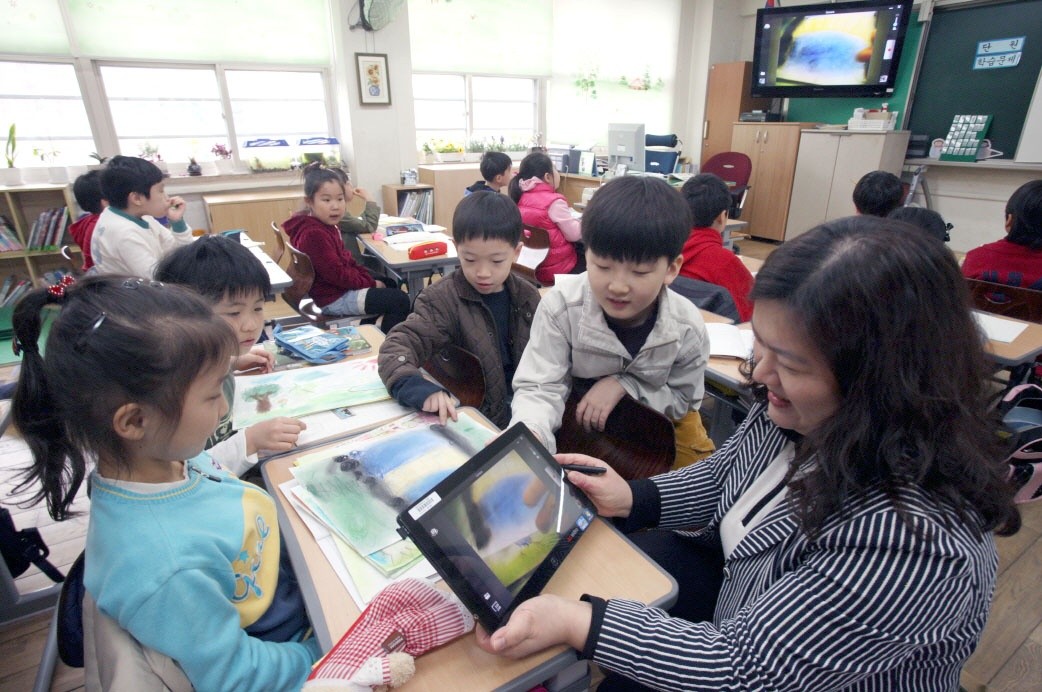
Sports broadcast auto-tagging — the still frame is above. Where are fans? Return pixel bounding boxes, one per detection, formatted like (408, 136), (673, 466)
(356, 0), (403, 32)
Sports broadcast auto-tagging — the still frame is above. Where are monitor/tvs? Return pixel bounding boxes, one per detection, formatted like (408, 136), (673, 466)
(748, 0), (915, 100)
(603, 121), (647, 178)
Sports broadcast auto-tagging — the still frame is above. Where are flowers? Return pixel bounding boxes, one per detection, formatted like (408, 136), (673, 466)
(436, 143), (464, 152)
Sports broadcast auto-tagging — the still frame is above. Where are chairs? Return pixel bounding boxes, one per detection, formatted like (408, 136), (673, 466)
(699, 151), (752, 254)
(644, 134), (684, 174)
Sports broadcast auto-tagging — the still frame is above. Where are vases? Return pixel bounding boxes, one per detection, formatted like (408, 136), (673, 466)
(438, 153), (464, 162)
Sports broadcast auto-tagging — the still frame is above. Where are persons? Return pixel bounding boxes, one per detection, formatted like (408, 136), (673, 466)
(14, 151), (1042, 692)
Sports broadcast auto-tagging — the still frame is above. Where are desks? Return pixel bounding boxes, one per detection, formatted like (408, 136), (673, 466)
(219, 212), (1042, 692)
(555, 172), (610, 213)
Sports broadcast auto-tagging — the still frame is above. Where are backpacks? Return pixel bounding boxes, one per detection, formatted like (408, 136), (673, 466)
(988, 384), (1042, 504)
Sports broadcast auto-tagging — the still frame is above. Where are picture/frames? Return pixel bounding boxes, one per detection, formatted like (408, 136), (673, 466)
(355, 52), (392, 106)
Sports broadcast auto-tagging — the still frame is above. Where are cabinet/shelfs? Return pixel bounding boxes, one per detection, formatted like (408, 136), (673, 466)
(202, 190), (309, 271)
(728, 125), (912, 247)
(700, 59), (773, 168)
(0, 183), (86, 307)
(381, 184), (434, 217)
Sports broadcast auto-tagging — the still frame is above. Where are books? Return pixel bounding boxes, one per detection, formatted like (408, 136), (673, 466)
(397, 191), (433, 225)
(0, 207), (68, 251)
(703, 323), (756, 361)
(0, 277), (31, 307)
(354, 212), (457, 252)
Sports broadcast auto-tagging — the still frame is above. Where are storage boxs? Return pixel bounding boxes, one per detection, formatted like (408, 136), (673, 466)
(244, 136), (342, 171)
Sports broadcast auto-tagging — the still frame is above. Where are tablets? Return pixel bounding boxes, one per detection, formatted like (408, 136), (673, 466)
(394, 421), (599, 638)
